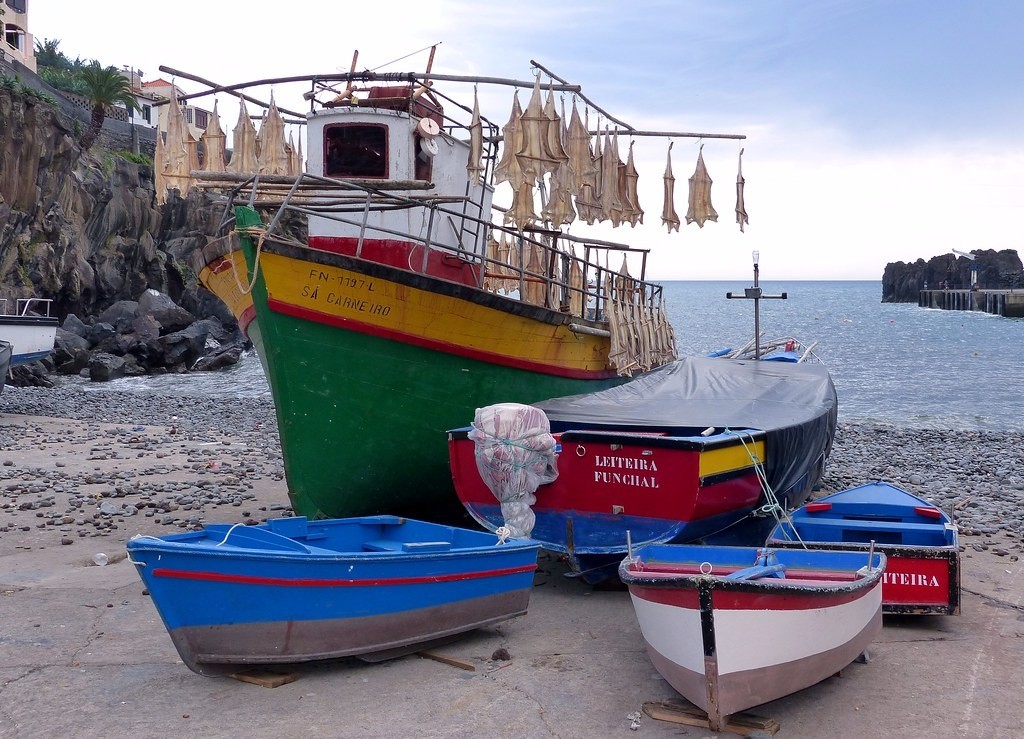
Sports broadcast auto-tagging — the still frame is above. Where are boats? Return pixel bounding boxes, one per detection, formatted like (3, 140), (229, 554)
(152, 44), (749, 520)
(443, 250), (838, 585)
(0, 298), (59, 369)
(0, 341), (12, 393)
(766, 477), (962, 619)
(619, 528), (887, 733)
(126, 514), (542, 678)
